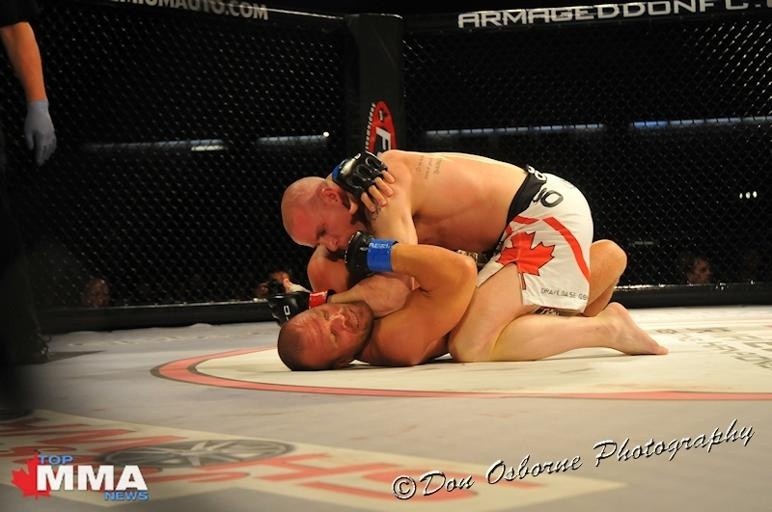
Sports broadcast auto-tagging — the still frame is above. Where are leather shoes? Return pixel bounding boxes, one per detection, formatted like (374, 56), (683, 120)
(17, 334), (50, 362)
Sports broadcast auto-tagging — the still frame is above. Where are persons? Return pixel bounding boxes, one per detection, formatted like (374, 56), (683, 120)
(264, 148), (669, 364)
(253, 259), (310, 324)
(675, 252), (726, 305)
(1, 1), (64, 366)
(274, 151), (632, 373)
(58, 274), (120, 332)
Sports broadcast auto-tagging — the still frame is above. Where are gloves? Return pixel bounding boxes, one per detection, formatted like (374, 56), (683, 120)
(24, 99), (57, 165)
